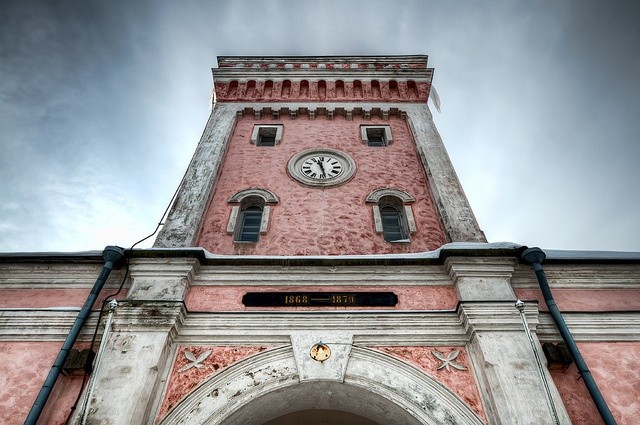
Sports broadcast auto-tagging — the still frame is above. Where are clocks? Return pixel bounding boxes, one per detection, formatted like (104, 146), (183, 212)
(285, 145), (357, 188)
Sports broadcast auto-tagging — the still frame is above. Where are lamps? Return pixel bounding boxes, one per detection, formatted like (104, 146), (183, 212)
(309, 339), (331, 363)
